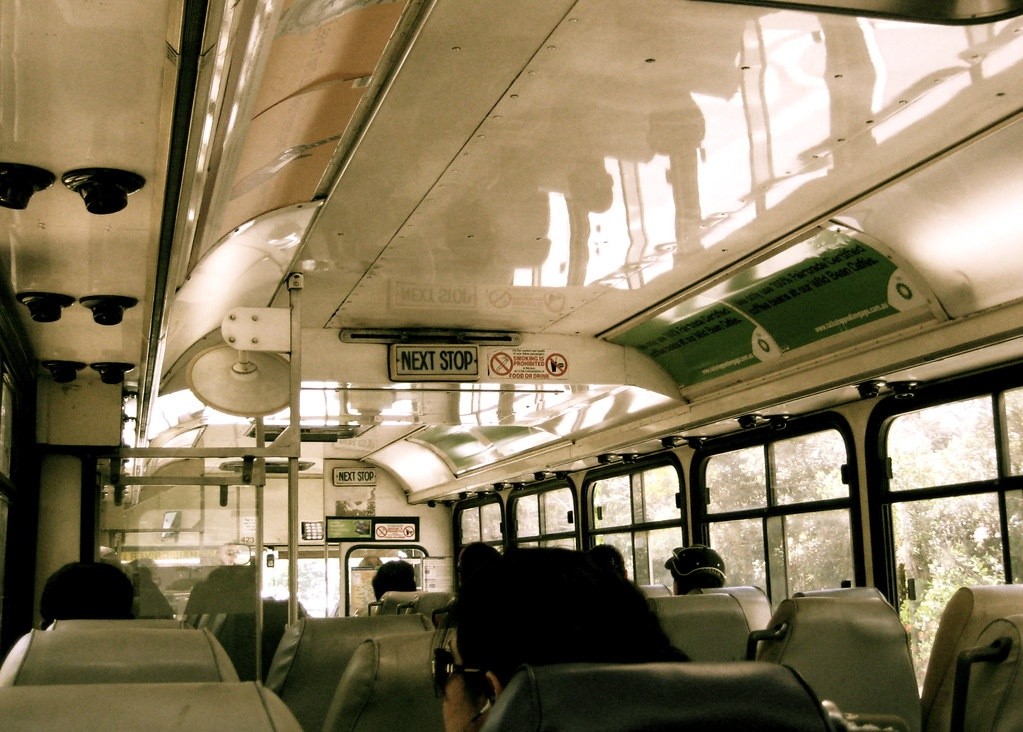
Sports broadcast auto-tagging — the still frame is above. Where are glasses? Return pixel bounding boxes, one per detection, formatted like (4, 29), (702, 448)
(432, 647), (483, 697)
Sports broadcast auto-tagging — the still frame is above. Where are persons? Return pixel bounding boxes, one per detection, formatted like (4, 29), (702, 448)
(431, 546), (692, 732)
(353, 560), (417, 617)
(40, 561), (136, 630)
(664, 544), (726, 594)
(589, 544), (627, 578)
(458, 541), (500, 583)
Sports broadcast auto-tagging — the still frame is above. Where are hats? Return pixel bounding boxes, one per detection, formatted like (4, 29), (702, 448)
(665, 545), (725, 579)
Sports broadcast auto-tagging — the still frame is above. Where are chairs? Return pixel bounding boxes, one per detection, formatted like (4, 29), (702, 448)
(0, 565), (1022, 732)
